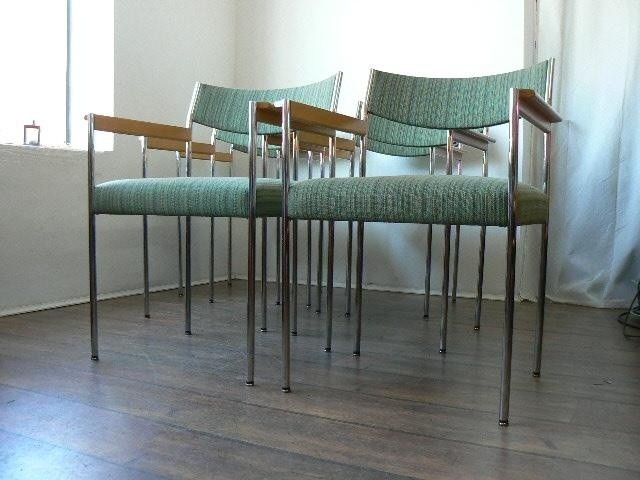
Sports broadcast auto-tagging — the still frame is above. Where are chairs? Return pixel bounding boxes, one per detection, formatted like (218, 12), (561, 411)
(281, 58), (565, 429)
(85, 69), (363, 390)
